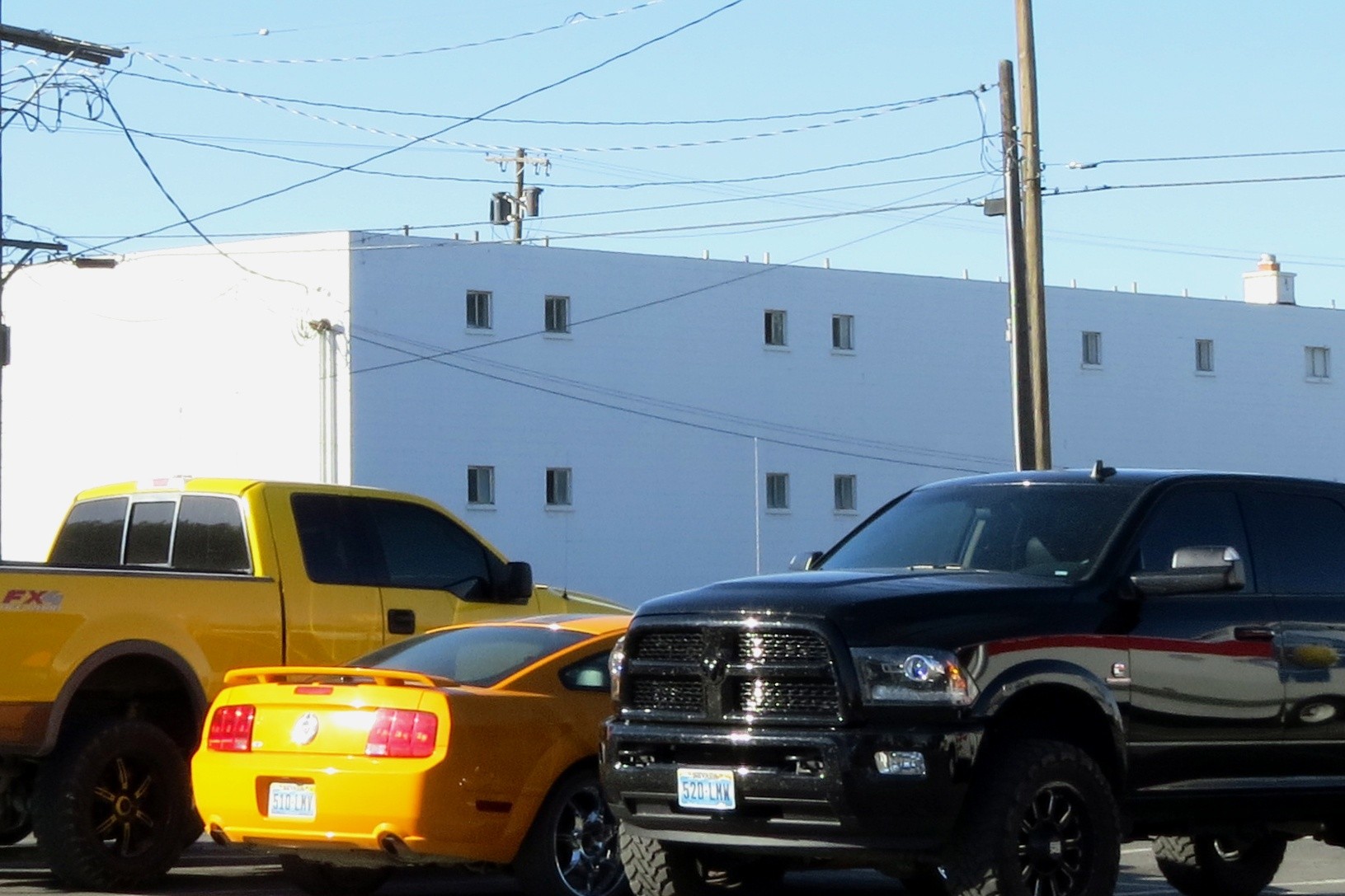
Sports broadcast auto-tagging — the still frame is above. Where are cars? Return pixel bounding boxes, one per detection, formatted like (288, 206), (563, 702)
(188, 610), (636, 896)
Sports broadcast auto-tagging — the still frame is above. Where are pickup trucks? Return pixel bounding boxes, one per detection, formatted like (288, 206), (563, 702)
(0, 477), (632, 890)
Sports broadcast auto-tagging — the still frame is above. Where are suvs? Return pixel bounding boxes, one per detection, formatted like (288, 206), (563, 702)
(592, 463), (1345, 895)
